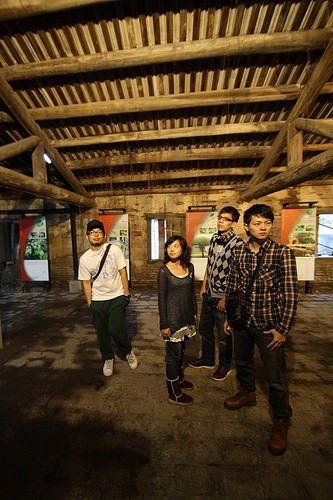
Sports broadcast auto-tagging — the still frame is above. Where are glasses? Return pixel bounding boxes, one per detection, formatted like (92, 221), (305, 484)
(217, 215), (233, 222)
(87, 230), (102, 236)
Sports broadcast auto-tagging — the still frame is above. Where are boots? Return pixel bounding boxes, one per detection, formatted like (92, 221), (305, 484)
(166, 376), (193, 405)
(224, 384), (258, 411)
(267, 417), (290, 456)
(178, 366), (194, 390)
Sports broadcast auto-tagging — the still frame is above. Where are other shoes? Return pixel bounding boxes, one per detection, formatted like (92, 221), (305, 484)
(102, 359), (115, 377)
(126, 347), (138, 371)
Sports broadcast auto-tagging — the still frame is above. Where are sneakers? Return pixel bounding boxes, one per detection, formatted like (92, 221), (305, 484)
(187, 358), (215, 370)
(212, 366), (231, 381)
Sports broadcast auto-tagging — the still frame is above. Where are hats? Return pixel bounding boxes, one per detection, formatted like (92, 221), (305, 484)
(87, 219), (104, 232)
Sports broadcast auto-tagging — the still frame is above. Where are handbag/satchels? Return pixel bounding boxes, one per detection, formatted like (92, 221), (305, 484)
(225, 251), (265, 330)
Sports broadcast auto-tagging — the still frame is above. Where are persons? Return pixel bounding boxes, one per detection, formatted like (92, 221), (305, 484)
(77, 220), (138, 376)
(158, 235), (198, 405)
(187, 206), (245, 381)
(224, 204), (299, 456)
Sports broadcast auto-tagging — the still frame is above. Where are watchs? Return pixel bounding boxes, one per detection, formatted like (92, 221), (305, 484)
(125, 294), (131, 299)
